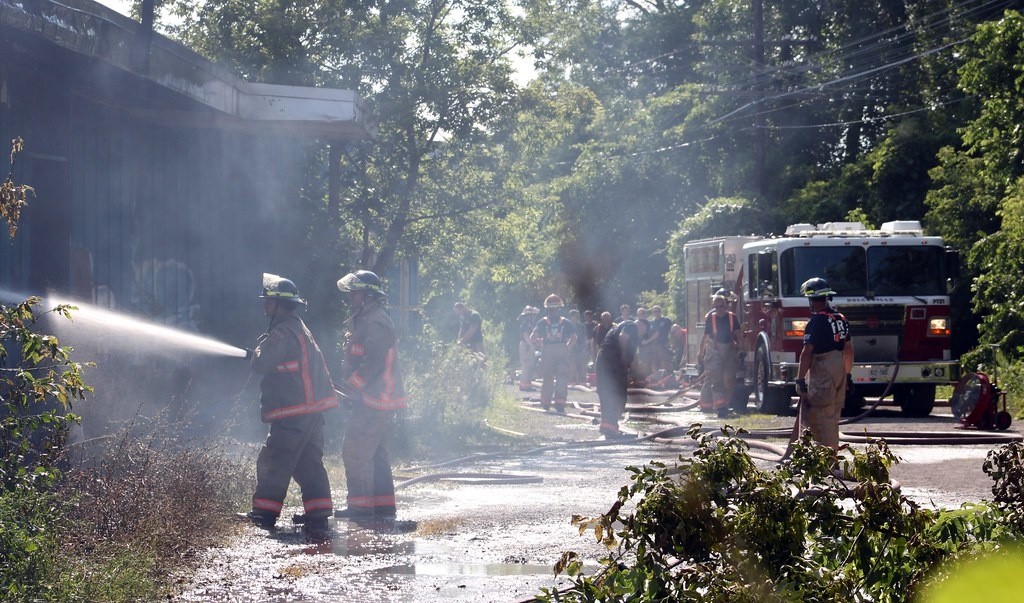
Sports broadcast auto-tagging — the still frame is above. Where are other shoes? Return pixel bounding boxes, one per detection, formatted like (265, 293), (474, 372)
(335, 505), (396, 517)
(542, 403), (565, 413)
(520, 386), (537, 393)
(599, 429), (626, 438)
(292, 507), (333, 524)
(699, 406), (731, 419)
(234, 508), (279, 526)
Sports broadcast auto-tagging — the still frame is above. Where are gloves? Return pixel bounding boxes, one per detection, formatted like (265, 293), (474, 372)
(796, 378), (809, 395)
(847, 373), (855, 388)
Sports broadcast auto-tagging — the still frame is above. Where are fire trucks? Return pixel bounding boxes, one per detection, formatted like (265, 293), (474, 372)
(679, 220), (963, 418)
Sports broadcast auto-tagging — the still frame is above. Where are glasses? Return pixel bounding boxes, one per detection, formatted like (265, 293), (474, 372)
(715, 303), (725, 309)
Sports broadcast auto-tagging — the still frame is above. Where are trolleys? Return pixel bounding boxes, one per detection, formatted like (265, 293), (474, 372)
(952, 343), (1012, 431)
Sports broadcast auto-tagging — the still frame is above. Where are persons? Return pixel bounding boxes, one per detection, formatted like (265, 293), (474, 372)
(454, 302), (483, 353)
(333, 271), (410, 517)
(235, 272), (340, 530)
(531, 293), (578, 412)
(698, 288), (746, 419)
(793, 278), (854, 452)
(595, 319), (650, 439)
(517, 304), (687, 391)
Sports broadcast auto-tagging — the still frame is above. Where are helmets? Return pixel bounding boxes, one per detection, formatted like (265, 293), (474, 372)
(260, 279), (304, 305)
(803, 279), (837, 297)
(346, 270), (387, 298)
(520, 305), (540, 316)
(544, 293), (564, 309)
